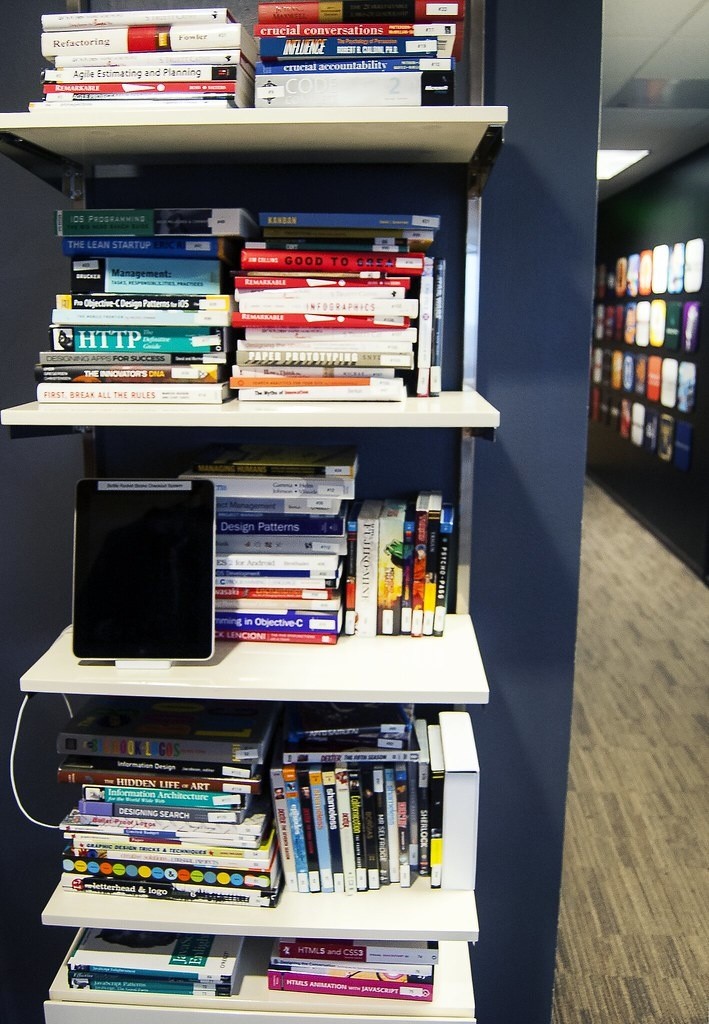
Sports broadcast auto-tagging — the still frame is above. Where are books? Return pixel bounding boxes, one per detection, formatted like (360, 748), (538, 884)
(29, 0), (481, 1001)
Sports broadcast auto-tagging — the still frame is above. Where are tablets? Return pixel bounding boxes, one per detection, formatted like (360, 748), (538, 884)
(72, 478), (216, 662)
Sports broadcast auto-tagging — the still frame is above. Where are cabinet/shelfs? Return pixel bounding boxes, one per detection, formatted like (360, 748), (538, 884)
(0, 97), (513, 1024)
